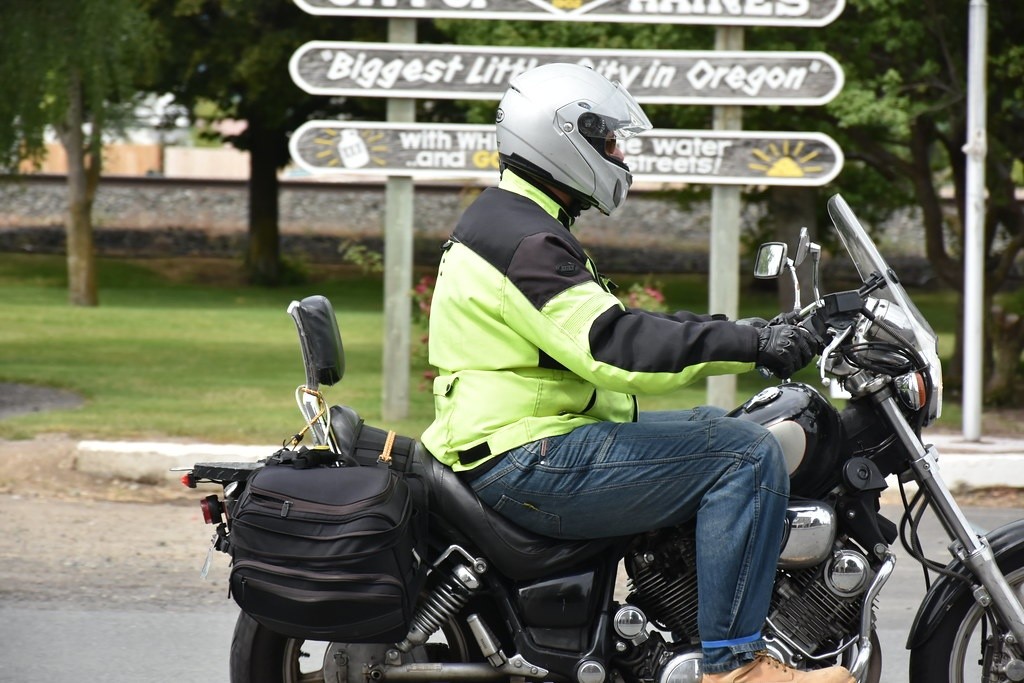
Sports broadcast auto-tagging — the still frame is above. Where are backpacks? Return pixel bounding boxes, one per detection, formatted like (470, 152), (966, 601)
(225, 459), (423, 646)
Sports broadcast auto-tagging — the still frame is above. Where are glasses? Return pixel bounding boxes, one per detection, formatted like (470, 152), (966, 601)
(597, 127), (619, 157)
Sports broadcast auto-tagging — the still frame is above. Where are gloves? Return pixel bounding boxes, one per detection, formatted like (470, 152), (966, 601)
(757, 325), (818, 379)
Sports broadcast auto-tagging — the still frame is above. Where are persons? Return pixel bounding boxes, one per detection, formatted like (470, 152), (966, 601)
(421, 63), (857, 683)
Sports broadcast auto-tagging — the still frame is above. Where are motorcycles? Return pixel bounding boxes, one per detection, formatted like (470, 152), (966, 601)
(171, 193), (1024, 683)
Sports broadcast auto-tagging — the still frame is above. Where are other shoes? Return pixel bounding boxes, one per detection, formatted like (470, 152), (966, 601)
(700, 650), (856, 682)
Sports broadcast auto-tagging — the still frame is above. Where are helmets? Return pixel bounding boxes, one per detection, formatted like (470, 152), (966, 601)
(494, 61), (654, 217)
